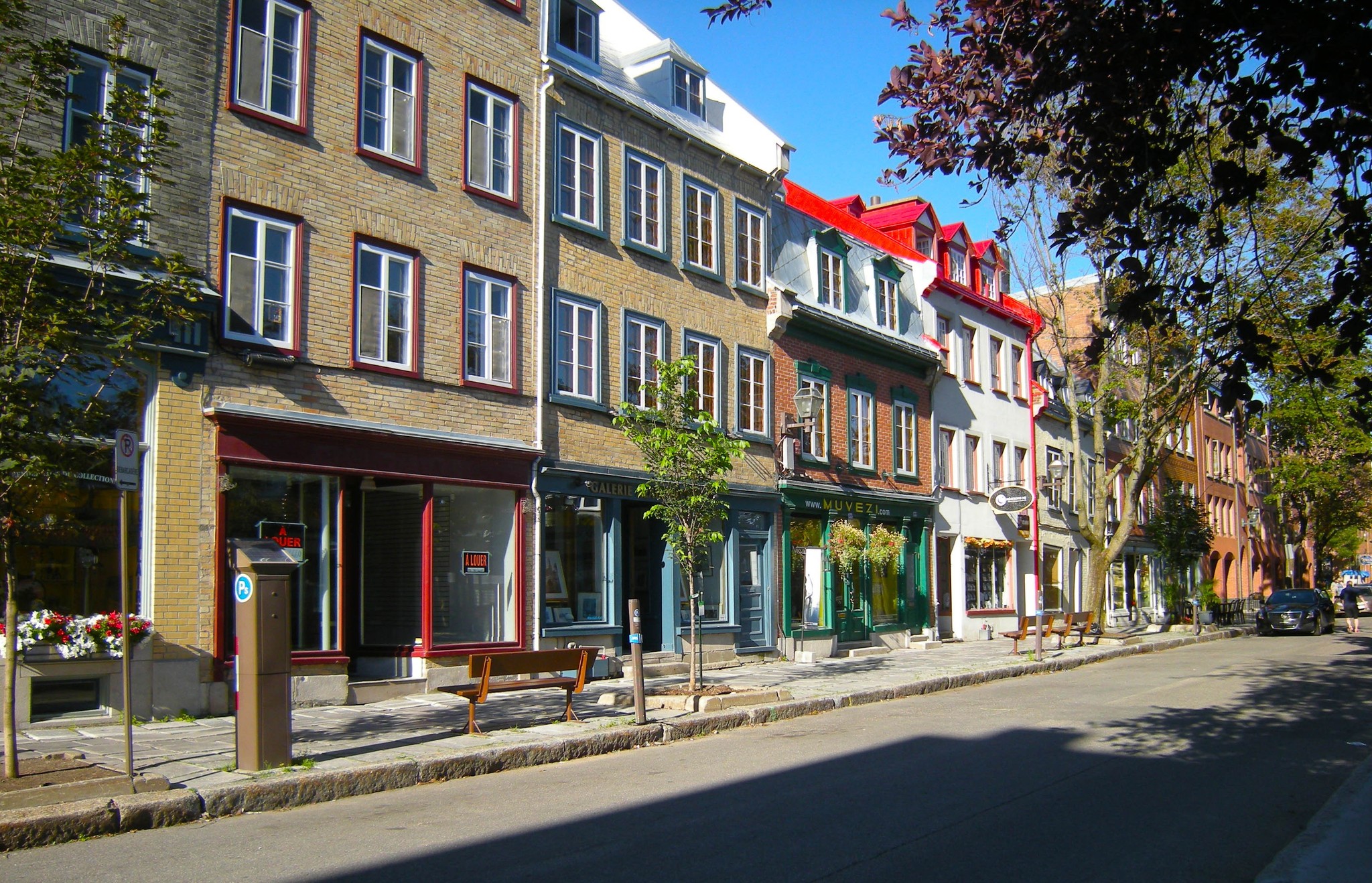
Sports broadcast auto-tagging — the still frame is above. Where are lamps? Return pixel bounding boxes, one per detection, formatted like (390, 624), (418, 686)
(780, 388), (824, 435)
(1039, 460), (1068, 490)
(1242, 508), (1258, 528)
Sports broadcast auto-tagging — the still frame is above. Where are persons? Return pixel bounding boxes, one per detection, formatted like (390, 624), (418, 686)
(1331, 577), (1344, 603)
(1341, 581), (1366, 633)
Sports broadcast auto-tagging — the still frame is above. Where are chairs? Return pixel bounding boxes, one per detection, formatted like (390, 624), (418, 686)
(1183, 599), (1246, 626)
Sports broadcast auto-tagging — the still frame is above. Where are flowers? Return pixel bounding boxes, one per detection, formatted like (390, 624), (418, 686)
(0, 609), (155, 660)
(827, 518), (908, 582)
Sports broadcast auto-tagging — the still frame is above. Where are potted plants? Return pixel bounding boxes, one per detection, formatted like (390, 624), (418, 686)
(1194, 577), (1222, 624)
(1155, 580), (1185, 624)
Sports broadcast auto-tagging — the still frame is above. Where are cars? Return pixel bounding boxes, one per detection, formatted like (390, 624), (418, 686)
(1255, 588), (1335, 637)
(1333, 583), (1372, 618)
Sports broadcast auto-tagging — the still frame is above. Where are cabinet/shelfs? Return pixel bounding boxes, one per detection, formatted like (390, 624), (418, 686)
(966, 563), (998, 610)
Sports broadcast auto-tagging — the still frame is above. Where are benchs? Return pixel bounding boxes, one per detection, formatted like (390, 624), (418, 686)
(437, 647), (599, 734)
(998, 611), (1094, 656)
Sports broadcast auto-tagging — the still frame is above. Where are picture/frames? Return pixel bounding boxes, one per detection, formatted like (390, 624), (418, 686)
(680, 545), (719, 622)
(546, 550), (602, 622)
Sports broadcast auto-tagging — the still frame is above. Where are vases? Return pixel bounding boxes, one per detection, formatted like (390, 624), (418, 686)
(21, 639), (134, 663)
